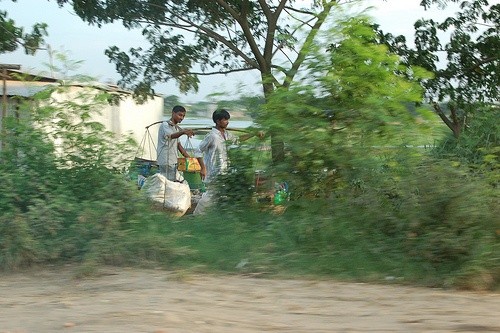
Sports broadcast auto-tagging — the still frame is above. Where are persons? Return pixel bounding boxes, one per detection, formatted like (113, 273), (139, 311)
(157, 105), (195, 183)
(194, 109), (265, 188)
